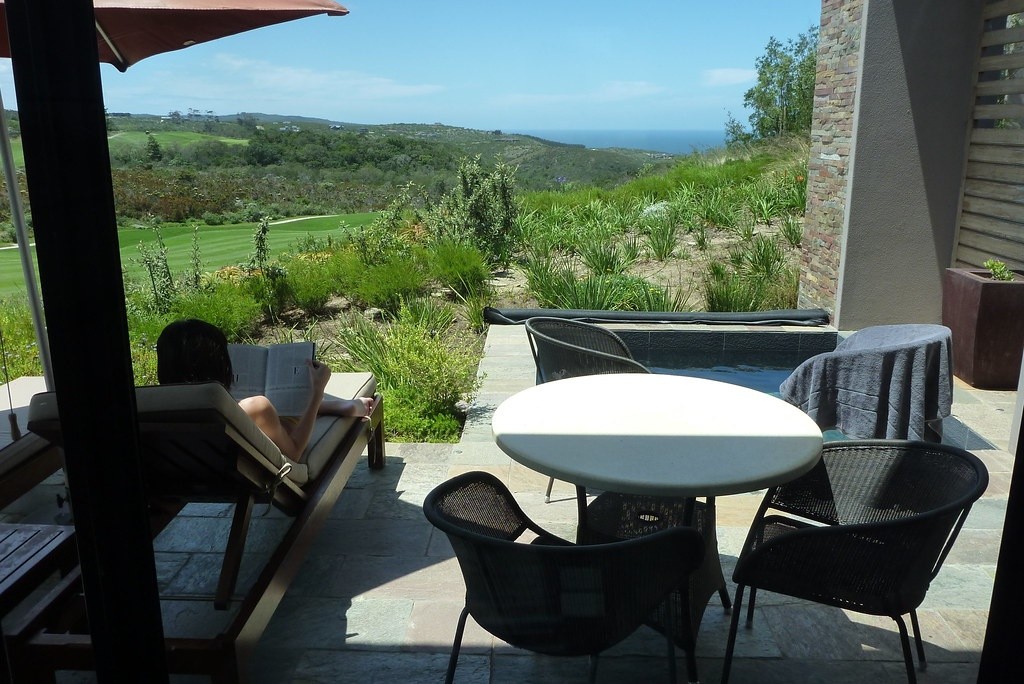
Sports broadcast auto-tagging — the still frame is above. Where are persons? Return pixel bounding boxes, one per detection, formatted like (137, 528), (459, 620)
(155, 320), (374, 464)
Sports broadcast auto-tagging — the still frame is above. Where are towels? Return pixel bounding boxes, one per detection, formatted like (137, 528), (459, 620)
(780, 323), (953, 444)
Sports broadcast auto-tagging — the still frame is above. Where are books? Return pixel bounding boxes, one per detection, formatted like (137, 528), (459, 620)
(223, 341), (317, 417)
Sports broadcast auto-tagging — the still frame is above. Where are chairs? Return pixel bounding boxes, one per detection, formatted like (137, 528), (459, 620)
(421, 470), (733, 684)
(779, 322), (955, 444)
(0, 371), (386, 684)
(524, 315), (651, 502)
(721, 439), (990, 684)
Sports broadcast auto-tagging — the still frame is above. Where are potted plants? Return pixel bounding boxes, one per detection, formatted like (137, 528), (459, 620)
(941, 256), (1024, 392)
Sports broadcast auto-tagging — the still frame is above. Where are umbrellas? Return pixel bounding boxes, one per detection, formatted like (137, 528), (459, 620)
(0, 0), (348, 395)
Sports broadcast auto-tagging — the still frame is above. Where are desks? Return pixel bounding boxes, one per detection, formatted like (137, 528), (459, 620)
(0, 523), (75, 595)
(490, 373), (826, 614)
